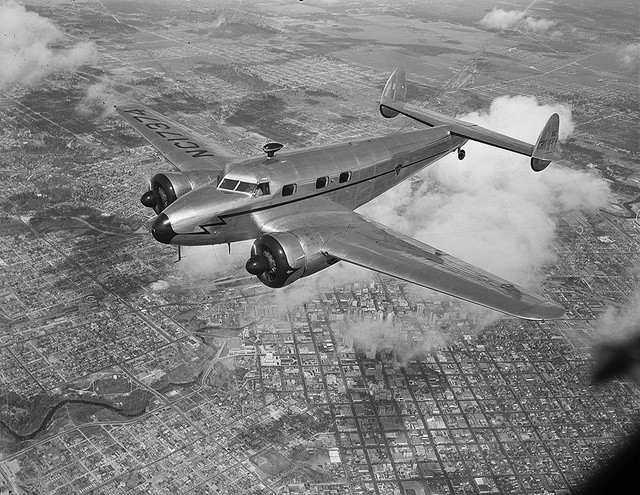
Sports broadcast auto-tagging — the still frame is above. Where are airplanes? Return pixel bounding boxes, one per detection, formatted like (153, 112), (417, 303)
(114, 66), (566, 323)
